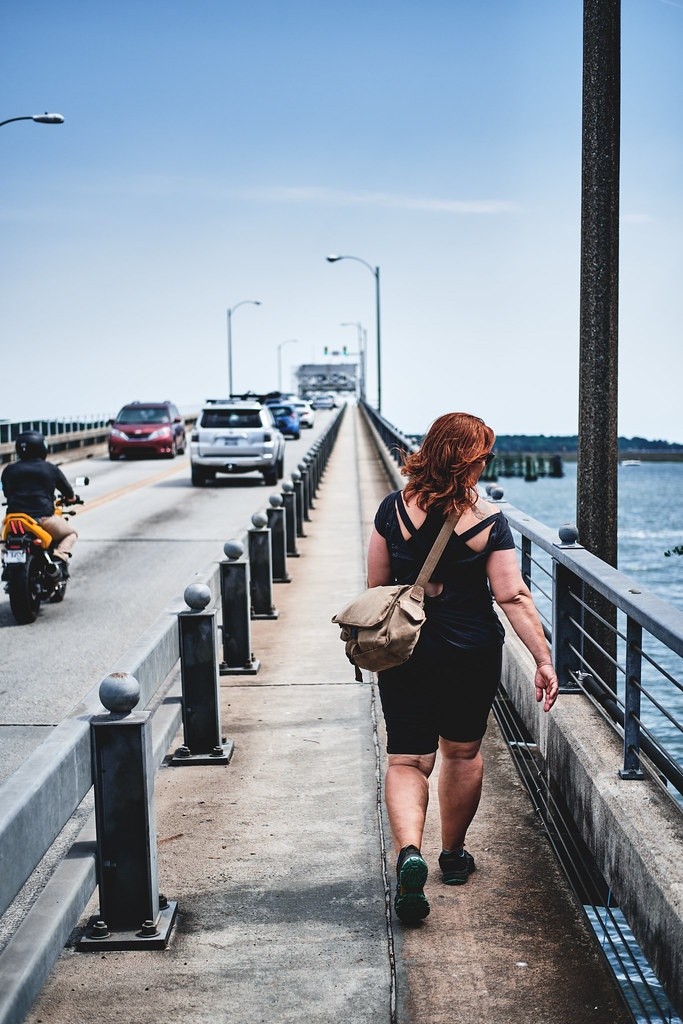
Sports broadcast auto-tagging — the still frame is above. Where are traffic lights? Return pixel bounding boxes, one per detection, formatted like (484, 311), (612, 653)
(324, 346), (346, 355)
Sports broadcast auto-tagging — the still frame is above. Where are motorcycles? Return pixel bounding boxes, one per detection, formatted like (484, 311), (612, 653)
(0, 476), (89, 625)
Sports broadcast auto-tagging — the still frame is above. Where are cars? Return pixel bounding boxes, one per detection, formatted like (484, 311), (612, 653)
(305, 391), (349, 411)
(267, 403), (301, 440)
(280, 399), (315, 429)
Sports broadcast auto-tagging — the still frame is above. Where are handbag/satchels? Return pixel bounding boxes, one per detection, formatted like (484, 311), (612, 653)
(332, 490), (472, 682)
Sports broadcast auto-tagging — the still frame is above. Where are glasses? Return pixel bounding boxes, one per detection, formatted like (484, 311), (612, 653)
(475, 452), (495, 463)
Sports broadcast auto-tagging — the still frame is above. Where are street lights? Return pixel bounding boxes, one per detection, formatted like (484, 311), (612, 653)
(340, 323), (367, 400)
(327, 256), (380, 414)
(227, 300), (260, 400)
(278, 340), (298, 393)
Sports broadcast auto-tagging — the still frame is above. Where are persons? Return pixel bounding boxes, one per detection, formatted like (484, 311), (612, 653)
(366, 412), (559, 924)
(1, 431), (79, 577)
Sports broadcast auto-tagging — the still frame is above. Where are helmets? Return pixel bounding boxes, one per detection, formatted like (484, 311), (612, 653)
(16, 431), (48, 461)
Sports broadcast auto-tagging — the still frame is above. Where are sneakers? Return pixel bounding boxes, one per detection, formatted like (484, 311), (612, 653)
(438, 849), (476, 886)
(393, 845), (430, 922)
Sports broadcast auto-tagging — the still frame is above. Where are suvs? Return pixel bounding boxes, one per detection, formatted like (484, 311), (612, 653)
(106, 400), (187, 461)
(189, 400), (286, 487)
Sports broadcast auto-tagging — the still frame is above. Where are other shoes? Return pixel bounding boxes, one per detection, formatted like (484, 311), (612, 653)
(44, 550), (70, 579)
